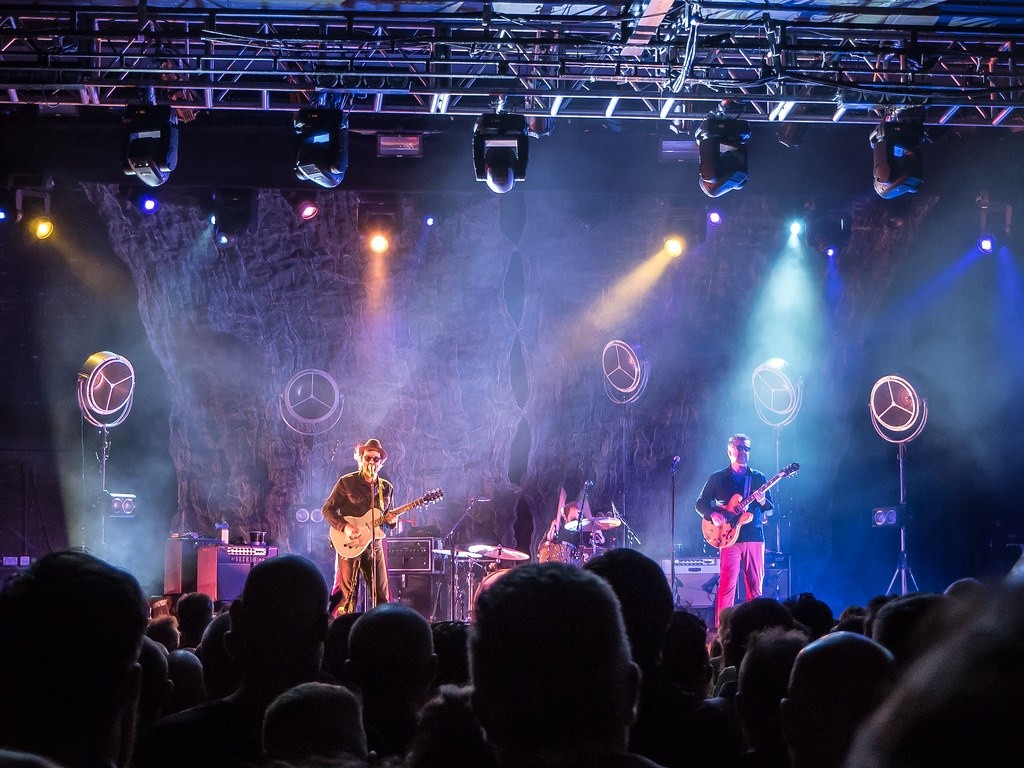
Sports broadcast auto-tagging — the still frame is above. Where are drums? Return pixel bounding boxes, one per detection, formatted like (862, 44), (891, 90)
(473, 568), (516, 617)
(538, 541), (577, 565)
(576, 546), (606, 566)
(564, 517), (622, 532)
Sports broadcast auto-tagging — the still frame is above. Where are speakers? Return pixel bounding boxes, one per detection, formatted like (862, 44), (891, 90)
(164, 538), (278, 605)
(737, 554), (791, 603)
(661, 556), (720, 610)
(94, 493), (136, 546)
(385, 536), (448, 623)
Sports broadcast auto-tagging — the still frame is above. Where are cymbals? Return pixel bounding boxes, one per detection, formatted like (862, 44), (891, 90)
(468, 544), (530, 561)
(431, 549), (482, 558)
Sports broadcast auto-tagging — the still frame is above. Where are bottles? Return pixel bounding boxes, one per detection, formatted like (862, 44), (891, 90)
(221, 520), (229, 545)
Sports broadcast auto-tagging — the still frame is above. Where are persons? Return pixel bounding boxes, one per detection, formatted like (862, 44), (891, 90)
(320, 439), (397, 613)
(696, 435), (775, 629)
(545, 502), (605, 566)
(0, 547), (1024, 768)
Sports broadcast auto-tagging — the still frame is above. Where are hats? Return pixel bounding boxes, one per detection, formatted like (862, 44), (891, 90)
(359, 439), (388, 463)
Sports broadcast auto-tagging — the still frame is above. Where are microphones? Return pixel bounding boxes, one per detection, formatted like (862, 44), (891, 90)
(671, 456), (680, 468)
(585, 480), (593, 485)
(367, 464), (375, 477)
(470, 497), (491, 502)
(612, 503), (619, 519)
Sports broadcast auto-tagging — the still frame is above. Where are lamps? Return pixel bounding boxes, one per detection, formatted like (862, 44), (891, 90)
(213, 192), (258, 247)
(695, 119), (752, 197)
(11, 189), (53, 240)
(356, 195), (403, 254)
(869, 121), (925, 199)
(294, 109), (349, 188)
(121, 99), (179, 186)
(976, 204), (1014, 255)
(805, 209), (852, 259)
(474, 113), (529, 193)
(663, 208), (706, 257)
(281, 191), (318, 220)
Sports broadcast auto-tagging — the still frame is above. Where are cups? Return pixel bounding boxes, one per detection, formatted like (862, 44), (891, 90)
(260, 531), (267, 545)
(250, 530), (261, 546)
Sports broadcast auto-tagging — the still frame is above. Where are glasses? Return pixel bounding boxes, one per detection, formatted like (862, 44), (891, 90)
(571, 517), (577, 521)
(731, 445), (751, 452)
(365, 456), (380, 462)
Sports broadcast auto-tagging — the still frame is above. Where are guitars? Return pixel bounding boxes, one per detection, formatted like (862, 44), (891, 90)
(329, 488), (444, 559)
(702, 462), (800, 548)
